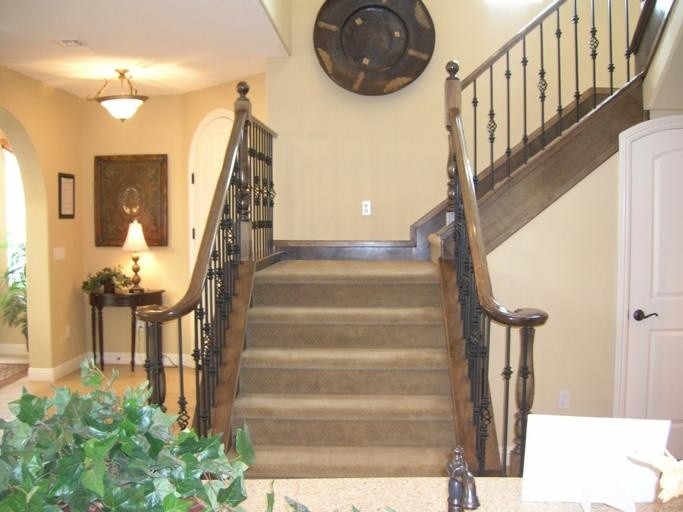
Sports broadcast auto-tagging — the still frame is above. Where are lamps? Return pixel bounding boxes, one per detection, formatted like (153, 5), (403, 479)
(120, 222), (149, 293)
(85, 68), (148, 124)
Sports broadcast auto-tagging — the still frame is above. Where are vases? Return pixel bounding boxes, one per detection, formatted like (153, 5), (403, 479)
(103, 281), (114, 294)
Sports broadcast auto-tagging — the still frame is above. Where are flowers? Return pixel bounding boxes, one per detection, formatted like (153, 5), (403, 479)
(81, 264), (132, 293)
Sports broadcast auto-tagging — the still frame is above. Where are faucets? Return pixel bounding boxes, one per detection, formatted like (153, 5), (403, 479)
(446, 445), (479, 512)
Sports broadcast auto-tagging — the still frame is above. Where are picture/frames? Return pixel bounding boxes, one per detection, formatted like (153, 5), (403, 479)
(91, 153), (168, 248)
(58, 172), (76, 220)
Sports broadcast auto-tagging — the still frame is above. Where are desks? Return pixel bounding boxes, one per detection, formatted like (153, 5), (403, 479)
(88, 289), (164, 373)
